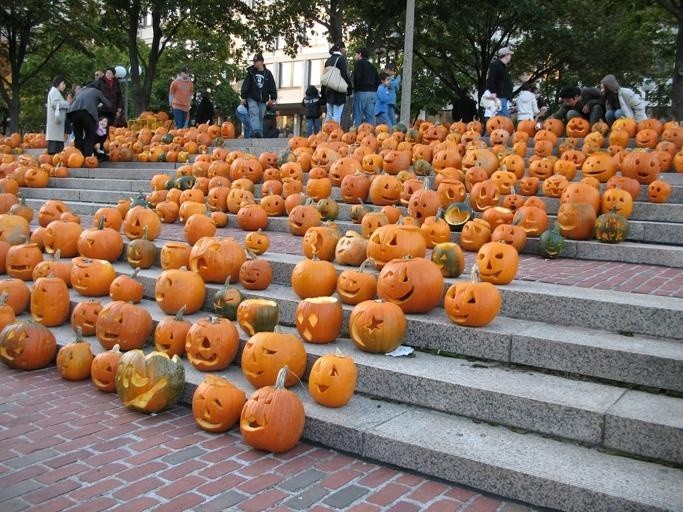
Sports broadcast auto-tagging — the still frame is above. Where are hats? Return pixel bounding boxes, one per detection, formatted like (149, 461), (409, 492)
(498, 48), (515, 57)
(602, 74), (618, 93)
(250, 54), (264, 61)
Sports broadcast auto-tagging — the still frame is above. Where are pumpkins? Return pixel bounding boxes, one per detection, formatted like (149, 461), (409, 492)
(165, 149), (178, 162)
(284, 193), (312, 219)
(291, 253), (337, 300)
(67, 153), (84, 168)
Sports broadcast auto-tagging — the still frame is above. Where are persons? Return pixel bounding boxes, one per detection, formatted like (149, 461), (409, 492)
(169, 66), (222, 129)
(453, 48), (648, 137)
(45, 67), (128, 161)
(302, 41), (401, 138)
(235, 54), (279, 138)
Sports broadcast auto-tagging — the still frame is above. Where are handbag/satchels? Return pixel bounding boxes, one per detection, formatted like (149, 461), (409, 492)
(55, 104), (62, 125)
(114, 111), (127, 128)
(321, 65), (348, 93)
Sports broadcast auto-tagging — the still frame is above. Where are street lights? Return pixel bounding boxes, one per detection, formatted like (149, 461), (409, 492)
(641, 77), (672, 119)
(113, 66), (141, 122)
